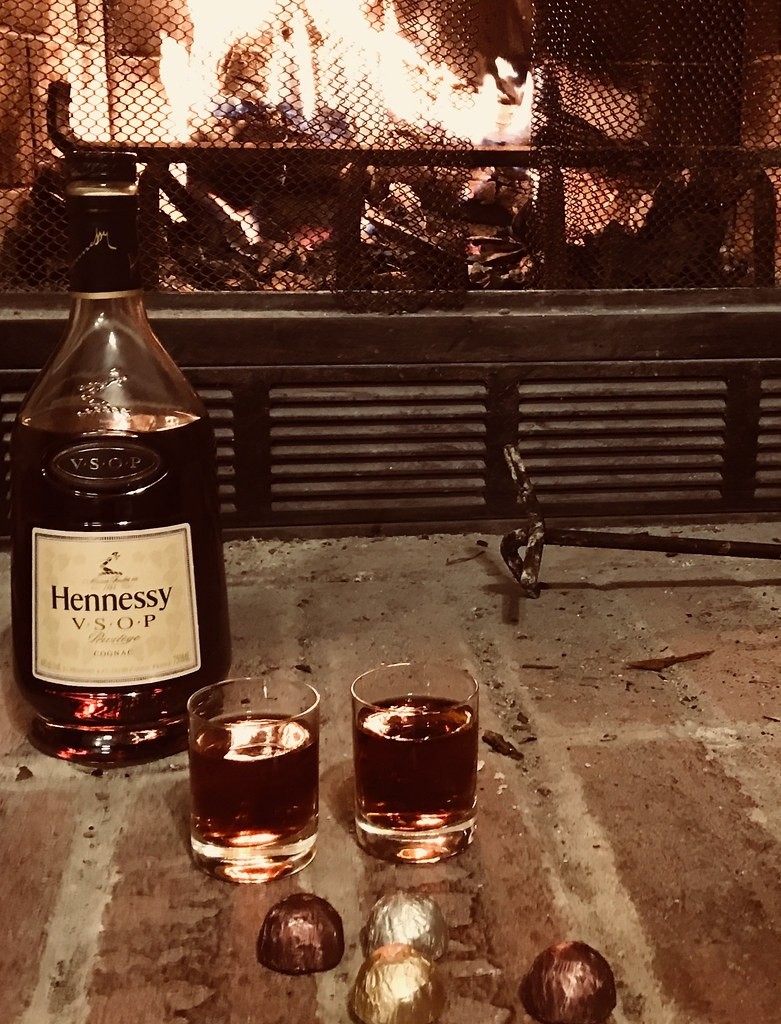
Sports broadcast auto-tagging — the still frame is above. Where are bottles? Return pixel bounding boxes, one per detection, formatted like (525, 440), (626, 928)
(2, 153), (234, 771)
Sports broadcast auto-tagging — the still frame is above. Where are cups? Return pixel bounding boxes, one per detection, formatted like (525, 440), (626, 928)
(350, 661), (480, 865)
(186, 676), (322, 885)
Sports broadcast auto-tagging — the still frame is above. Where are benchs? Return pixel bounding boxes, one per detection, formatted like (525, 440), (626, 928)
(0, 306), (781, 534)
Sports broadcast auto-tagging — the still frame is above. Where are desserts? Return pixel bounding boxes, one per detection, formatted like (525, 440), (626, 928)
(256, 886), (618, 1024)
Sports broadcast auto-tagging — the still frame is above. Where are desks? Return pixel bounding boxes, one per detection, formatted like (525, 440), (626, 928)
(0, 521), (781, 1024)
